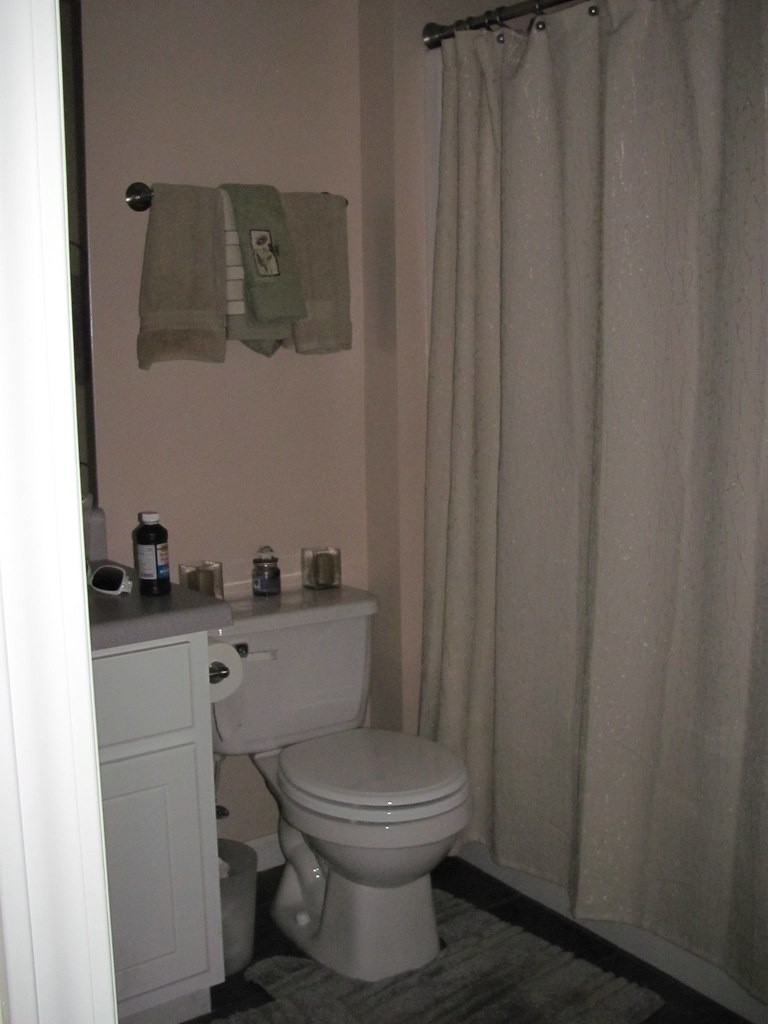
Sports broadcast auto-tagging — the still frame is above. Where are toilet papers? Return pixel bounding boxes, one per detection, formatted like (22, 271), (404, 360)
(206, 634), (245, 702)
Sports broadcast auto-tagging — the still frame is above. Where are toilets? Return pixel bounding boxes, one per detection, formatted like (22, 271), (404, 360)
(207, 582), (470, 982)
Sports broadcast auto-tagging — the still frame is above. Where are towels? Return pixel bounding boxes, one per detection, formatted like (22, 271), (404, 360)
(223, 191), (245, 315)
(221, 180), (310, 358)
(285, 193), (353, 356)
(134, 183), (227, 370)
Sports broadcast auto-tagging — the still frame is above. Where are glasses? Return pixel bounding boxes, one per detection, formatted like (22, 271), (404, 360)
(86, 560), (133, 595)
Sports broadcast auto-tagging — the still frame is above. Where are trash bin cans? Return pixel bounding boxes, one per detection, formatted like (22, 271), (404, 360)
(217, 837), (259, 980)
